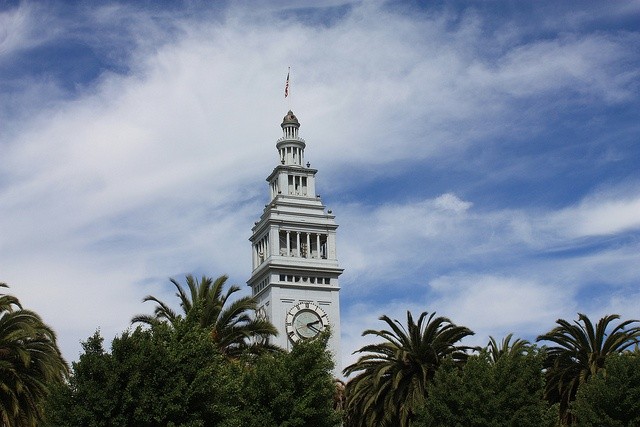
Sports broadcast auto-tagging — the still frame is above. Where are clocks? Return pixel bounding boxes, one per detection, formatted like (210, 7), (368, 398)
(285, 300), (330, 351)
